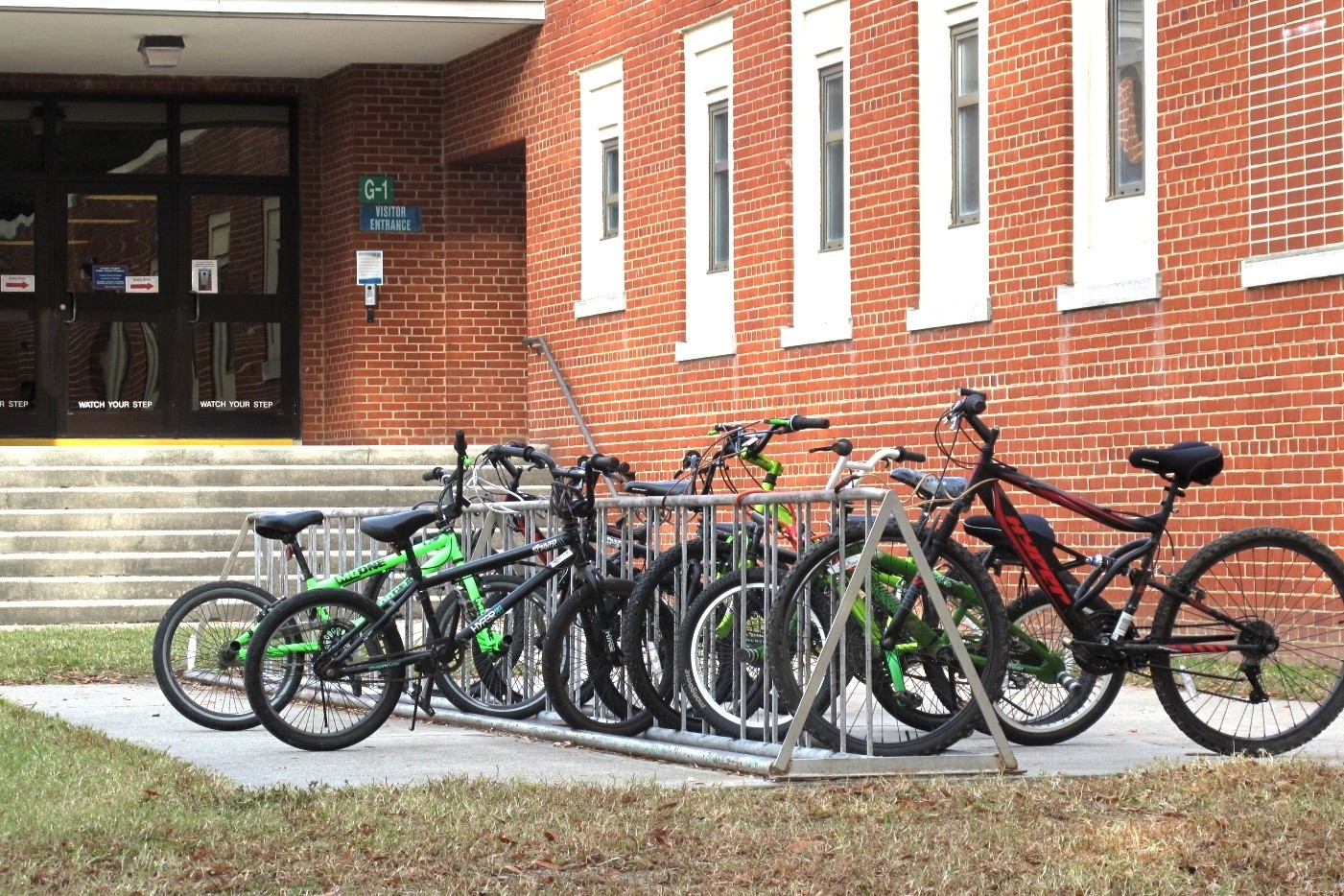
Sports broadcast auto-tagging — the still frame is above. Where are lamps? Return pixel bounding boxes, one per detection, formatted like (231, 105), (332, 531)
(136, 36), (186, 68)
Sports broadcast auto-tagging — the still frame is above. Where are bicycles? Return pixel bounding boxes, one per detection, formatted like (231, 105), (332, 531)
(151, 411), (1127, 756)
(763, 384), (1344, 760)
